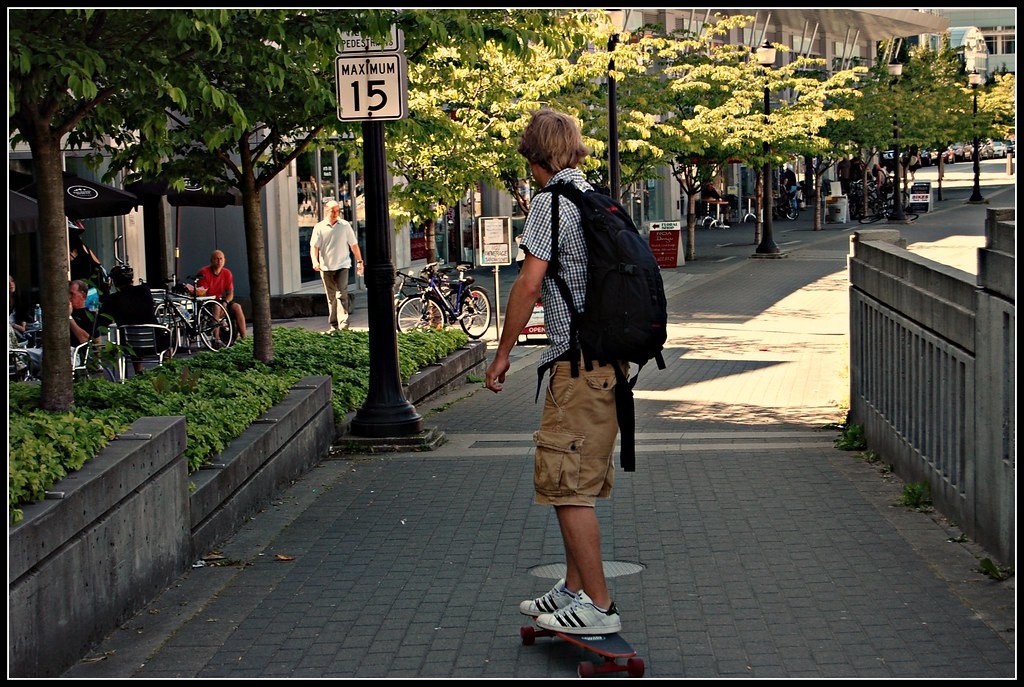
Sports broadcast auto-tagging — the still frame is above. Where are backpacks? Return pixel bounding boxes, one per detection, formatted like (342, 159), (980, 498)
(549, 179), (668, 373)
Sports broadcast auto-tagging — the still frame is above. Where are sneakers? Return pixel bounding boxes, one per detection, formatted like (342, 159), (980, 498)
(536, 589), (622, 634)
(519, 578), (581, 617)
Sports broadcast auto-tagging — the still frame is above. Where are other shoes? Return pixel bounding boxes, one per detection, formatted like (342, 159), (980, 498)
(211, 337), (227, 348)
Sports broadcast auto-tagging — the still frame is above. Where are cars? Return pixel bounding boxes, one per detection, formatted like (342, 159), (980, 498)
(884, 138), (995, 165)
(1004, 140), (1015, 158)
(992, 142), (1008, 157)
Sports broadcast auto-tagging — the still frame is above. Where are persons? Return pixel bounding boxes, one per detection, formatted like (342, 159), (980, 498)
(310, 201), (364, 333)
(9, 242), (163, 378)
(293, 174), (365, 216)
(700, 180), (723, 224)
(781, 145), (920, 220)
(486, 109), (631, 635)
(196, 249), (248, 347)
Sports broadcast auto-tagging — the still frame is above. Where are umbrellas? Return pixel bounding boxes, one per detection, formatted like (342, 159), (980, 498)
(10, 160), (240, 284)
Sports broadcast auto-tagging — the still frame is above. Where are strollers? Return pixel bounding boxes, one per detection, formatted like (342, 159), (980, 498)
(772, 190), (799, 221)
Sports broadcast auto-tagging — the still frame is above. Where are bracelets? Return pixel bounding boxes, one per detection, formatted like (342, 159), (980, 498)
(357, 260), (363, 263)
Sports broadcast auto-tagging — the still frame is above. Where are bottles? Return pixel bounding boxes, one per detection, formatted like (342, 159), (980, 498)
(440, 257), (444, 265)
(34, 304), (41, 324)
(181, 308), (191, 319)
(357, 260), (363, 276)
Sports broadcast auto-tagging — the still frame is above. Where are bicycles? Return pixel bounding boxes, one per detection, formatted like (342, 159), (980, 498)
(154, 279), (234, 360)
(394, 263), (492, 340)
(848, 170), (919, 224)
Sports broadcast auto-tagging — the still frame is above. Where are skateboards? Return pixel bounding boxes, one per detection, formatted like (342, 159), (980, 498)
(520, 613), (646, 678)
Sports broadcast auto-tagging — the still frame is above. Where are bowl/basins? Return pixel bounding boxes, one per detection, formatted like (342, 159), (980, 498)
(190, 290), (207, 296)
